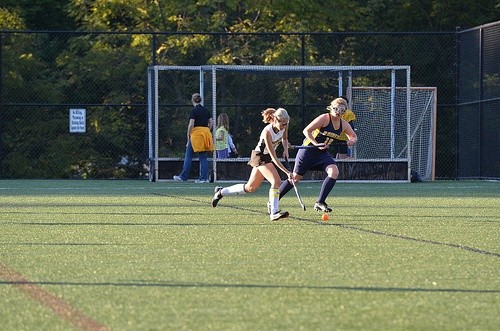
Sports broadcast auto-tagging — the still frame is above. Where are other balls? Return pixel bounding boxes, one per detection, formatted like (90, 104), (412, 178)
(322, 213), (328, 221)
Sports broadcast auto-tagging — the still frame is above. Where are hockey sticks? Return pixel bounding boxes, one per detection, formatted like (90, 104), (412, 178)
(287, 141), (357, 149)
(292, 178), (306, 212)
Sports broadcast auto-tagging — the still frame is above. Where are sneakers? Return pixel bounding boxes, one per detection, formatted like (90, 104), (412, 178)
(212, 187), (223, 207)
(314, 201), (332, 212)
(270, 211), (289, 221)
(268, 201), (271, 214)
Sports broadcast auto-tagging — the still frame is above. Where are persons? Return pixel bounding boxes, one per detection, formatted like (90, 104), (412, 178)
(172, 93), (213, 183)
(266, 98), (358, 215)
(208, 107), (237, 182)
(330, 97), (357, 160)
(211, 108), (294, 221)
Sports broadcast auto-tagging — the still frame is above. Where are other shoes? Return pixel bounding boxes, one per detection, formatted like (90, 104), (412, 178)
(195, 179), (209, 183)
(173, 176), (182, 180)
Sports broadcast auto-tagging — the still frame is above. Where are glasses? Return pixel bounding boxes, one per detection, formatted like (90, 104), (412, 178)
(280, 122), (289, 126)
(333, 107), (345, 114)
